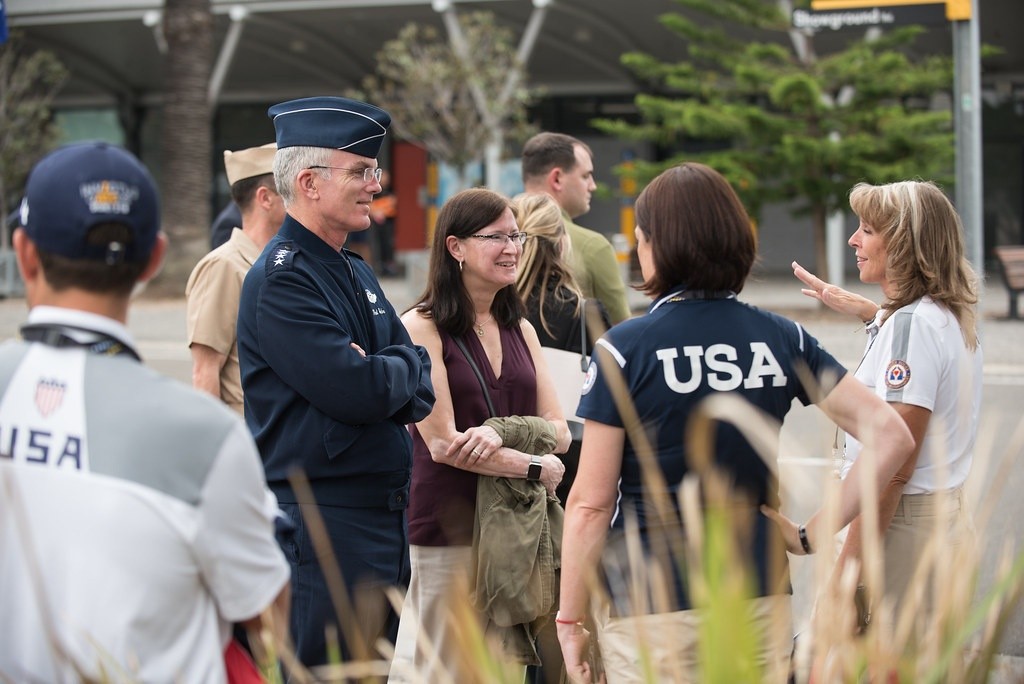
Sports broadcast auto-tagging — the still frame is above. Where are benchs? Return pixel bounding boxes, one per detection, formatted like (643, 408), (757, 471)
(991, 245), (1024, 319)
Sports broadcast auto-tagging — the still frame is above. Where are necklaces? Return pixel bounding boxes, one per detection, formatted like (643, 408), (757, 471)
(471, 312), (491, 336)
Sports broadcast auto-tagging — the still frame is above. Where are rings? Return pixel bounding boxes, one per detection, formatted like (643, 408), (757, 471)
(473, 450), (480, 455)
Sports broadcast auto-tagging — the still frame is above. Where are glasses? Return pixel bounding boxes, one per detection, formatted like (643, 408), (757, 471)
(306, 165), (383, 183)
(457, 232), (527, 246)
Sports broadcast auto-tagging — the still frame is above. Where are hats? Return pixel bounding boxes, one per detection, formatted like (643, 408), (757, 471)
(268, 96), (392, 159)
(8, 142), (160, 263)
(224, 142), (279, 186)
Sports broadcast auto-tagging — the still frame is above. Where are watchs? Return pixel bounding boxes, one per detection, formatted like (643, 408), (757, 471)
(526, 455), (544, 482)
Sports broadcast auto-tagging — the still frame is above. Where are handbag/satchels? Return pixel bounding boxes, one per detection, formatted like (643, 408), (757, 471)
(541, 299), (595, 443)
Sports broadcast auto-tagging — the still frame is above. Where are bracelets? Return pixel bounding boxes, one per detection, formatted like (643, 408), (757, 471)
(799, 522), (815, 555)
(555, 610), (586, 625)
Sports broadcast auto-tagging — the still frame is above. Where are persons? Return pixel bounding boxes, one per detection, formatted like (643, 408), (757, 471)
(513, 132), (634, 327)
(237, 96), (438, 683)
(495, 191), (593, 510)
(210, 200), (243, 250)
(793, 179), (979, 632)
(555, 162), (916, 684)
(387, 188), (573, 683)
(185, 142), (286, 419)
(0, 143), (292, 684)
